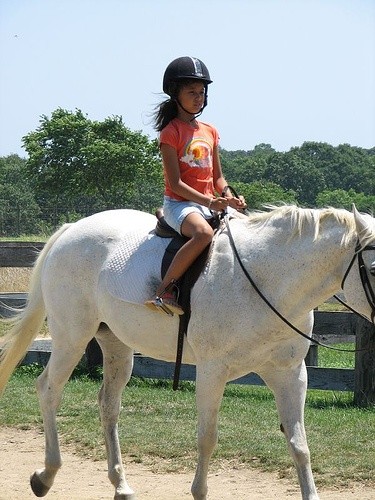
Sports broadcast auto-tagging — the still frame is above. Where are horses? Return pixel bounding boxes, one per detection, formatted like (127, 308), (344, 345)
(0, 200), (375, 500)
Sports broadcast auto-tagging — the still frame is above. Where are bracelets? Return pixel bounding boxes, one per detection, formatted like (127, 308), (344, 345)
(208, 198), (213, 210)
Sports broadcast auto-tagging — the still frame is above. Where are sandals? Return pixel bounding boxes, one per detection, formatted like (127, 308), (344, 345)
(145, 294), (185, 315)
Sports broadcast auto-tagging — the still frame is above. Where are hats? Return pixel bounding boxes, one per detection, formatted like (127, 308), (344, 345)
(163, 56), (213, 121)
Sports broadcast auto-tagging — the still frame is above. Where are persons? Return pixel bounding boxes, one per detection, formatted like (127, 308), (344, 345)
(144, 56), (246, 315)
